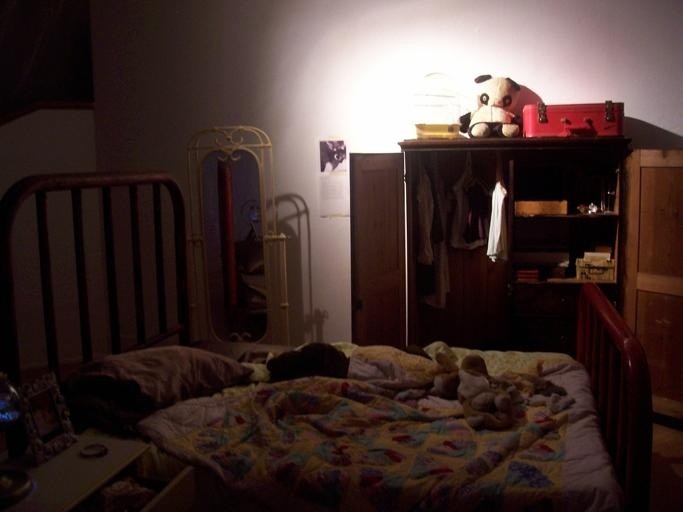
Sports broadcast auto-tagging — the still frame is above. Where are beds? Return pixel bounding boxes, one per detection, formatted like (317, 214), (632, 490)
(0, 172), (653, 512)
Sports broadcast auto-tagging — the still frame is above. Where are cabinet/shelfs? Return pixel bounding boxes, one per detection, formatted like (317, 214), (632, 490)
(350, 136), (683, 422)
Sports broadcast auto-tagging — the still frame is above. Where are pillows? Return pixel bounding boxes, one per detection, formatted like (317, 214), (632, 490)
(41, 344), (255, 414)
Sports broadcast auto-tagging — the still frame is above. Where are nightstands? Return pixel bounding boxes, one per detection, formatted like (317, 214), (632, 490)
(0, 432), (195, 512)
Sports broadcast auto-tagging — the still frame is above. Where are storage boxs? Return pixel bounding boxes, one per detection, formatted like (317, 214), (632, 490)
(575, 258), (616, 281)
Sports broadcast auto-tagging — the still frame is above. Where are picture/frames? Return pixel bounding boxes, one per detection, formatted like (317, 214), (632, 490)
(16, 371), (78, 467)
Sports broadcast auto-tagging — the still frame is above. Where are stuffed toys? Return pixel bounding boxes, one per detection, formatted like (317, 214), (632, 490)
(456, 73), (524, 138)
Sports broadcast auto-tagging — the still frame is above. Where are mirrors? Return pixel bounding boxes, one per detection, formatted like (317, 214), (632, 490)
(186, 125), (290, 347)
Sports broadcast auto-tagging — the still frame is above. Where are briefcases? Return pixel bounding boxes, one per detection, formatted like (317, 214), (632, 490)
(522, 101), (624, 138)
